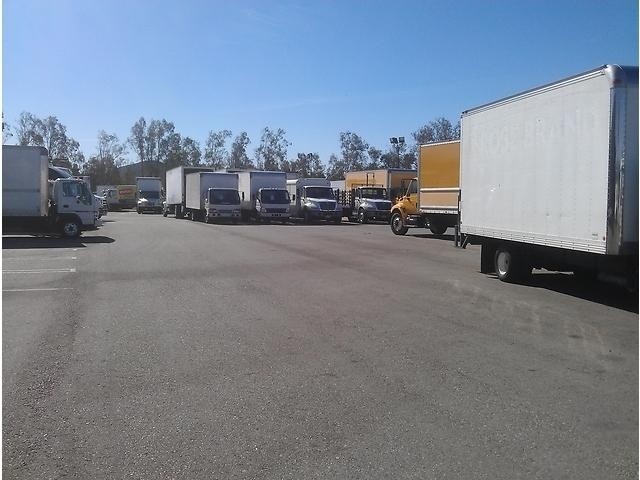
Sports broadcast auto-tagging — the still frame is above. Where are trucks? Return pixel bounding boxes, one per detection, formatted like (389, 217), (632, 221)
(334, 185), (392, 224)
(388, 139), (461, 236)
(185, 172), (243, 224)
(285, 177), (344, 225)
(136, 176), (163, 215)
(162, 166), (215, 218)
(344, 168), (418, 205)
(2, 142), (137, 240)
(233, 170), (292, 224)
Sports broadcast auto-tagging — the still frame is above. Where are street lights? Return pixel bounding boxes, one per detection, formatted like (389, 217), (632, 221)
(389, 137), (405, 167)
